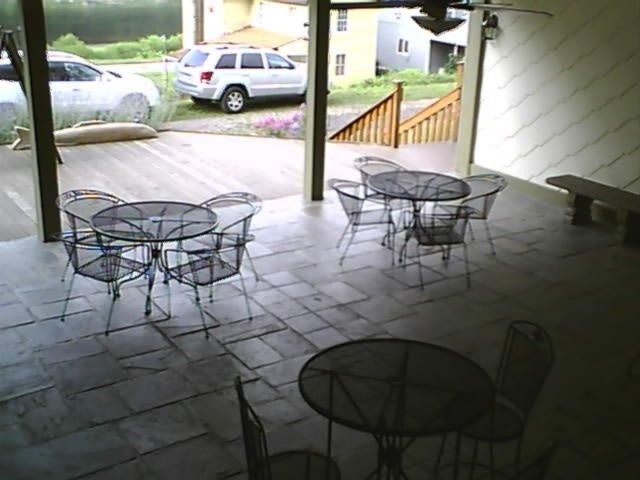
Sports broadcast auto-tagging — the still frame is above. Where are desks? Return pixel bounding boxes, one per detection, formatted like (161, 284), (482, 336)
(298, 339), (495, 480)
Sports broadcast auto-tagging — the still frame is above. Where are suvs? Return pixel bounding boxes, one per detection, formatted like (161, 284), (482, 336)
(173, 42), (331, 114)
(0, 48), (162, 140)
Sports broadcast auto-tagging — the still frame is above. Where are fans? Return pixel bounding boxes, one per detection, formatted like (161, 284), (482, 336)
(329, 0), (553, 35)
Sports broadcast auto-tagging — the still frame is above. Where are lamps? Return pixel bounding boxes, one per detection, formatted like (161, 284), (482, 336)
(482, 15), (498, 41)
(410, 5), (468, 37)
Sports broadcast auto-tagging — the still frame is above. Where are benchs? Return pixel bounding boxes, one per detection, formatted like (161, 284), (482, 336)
(546, 173), (640, 242)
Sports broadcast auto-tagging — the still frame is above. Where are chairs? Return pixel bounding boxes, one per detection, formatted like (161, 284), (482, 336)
(326, 156), (509, 291)
(478, 439), (561, 479)
(51, 156), (262, 344)
(432, 320), (553, 480)
(234, 375), (340, 479)
